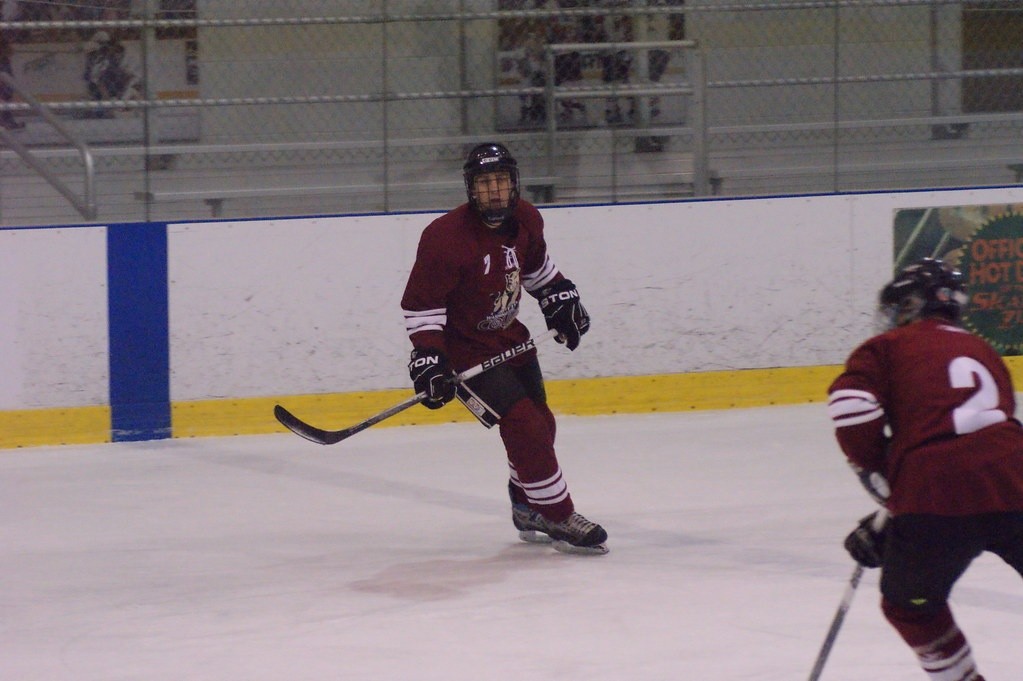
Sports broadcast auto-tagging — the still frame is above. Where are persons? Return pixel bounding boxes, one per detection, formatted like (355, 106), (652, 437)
(827, 257), (1023, 681)
(0, 0), (144, 132)
(399, 144), (611, 554)
(496, 1), (684, 153)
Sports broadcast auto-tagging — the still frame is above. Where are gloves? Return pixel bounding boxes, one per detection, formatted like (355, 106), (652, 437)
(539, 279), (591, 352)
(848, 460), (892, 508)
(408, 347), (457, 409)
(844, 509), (893, 569)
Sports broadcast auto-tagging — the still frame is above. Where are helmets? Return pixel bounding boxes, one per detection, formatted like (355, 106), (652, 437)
(462, 142), (520, 226)
(879, 257), (968, 328)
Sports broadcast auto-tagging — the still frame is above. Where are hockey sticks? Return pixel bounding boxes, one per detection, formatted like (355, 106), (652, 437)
(272, 314), (592, 445)
(809, 505), (890, 681)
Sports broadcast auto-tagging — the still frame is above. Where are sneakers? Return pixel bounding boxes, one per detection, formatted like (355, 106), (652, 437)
(545, 511), (609, 555)
(508, 479), (554, 543)
